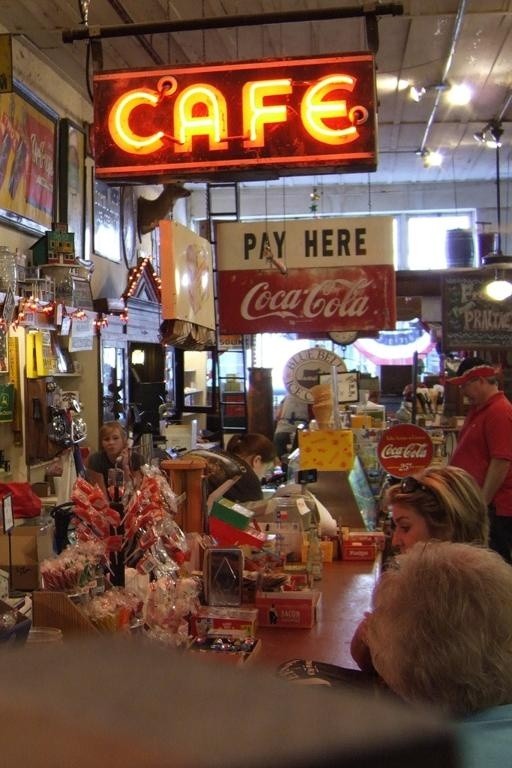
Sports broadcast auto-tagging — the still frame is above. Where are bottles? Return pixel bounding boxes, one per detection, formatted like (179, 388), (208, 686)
(305, 526), (322, 581)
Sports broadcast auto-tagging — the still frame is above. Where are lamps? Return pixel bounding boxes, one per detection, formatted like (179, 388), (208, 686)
(472, 118), (504, 148)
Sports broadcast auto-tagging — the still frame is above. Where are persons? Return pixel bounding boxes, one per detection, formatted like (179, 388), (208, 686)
(213, 431), (278, 502)
(442, 358), (512, 564)
(86, 420), (146, 503)
(270, 395), (313, 458)
(375, 538), (511, 766)
(350, 462), (489, 693)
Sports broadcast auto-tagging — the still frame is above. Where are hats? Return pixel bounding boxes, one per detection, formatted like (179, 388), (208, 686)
(447, 357), (495, 384)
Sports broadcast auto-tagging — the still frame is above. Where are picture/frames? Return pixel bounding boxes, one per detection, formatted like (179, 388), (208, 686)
(0, 69), (127, 269)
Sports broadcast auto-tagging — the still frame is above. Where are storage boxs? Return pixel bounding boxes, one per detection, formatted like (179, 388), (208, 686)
(252, 585), (322, 630)
(0, 525), (54, 591)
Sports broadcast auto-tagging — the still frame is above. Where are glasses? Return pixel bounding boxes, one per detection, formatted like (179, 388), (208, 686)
(401, 477), (429, 493)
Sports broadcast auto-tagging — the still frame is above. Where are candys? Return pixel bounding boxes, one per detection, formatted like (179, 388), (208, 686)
(41, 477), (254, 651)
